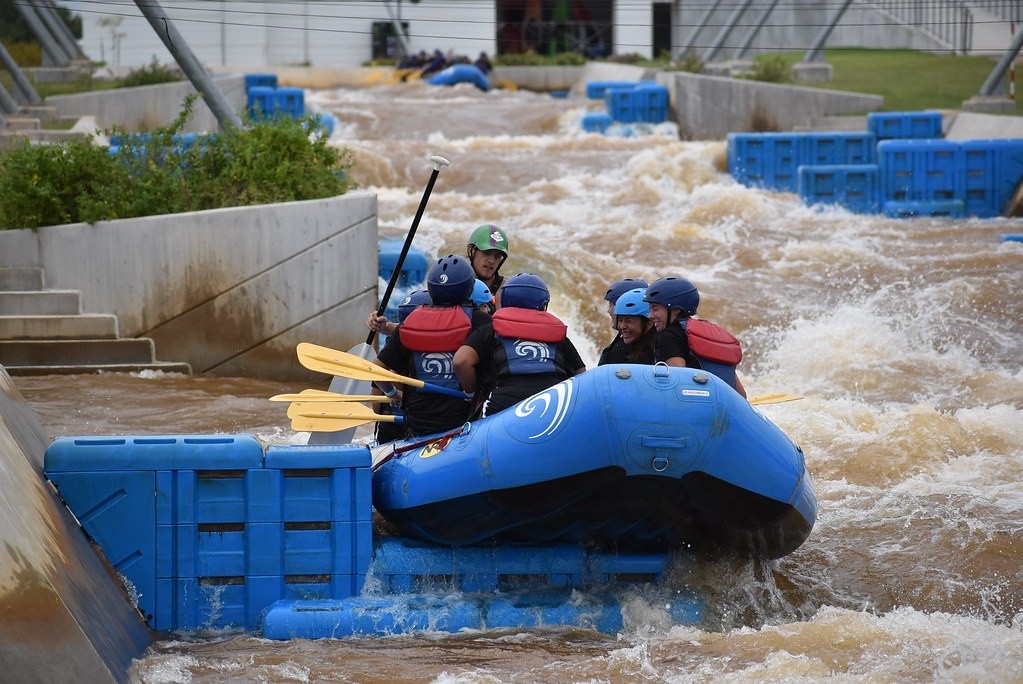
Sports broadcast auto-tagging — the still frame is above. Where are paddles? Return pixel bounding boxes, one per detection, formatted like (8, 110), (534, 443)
(308, 156), (450, 447)
(269, 342), (466, 434)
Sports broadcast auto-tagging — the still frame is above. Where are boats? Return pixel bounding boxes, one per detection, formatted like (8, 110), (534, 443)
(425, 63), (490, 94)
(370, 363), (819, 561)
(108, 113), (336, 171)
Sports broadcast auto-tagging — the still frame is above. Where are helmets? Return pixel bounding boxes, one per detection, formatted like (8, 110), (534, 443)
(604, 278), (649, 306)
(398, 289), (433, 321)
(467, 224), (509, 258)
(612, 288), (651, 320)
(501, 273), (550, 310)
(643, 277), (699, 316)
(428, 254), (476, 306)
(469, 279), (493, 307)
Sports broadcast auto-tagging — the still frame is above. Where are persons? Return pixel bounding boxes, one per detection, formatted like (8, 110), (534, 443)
(454, 272), (587, 421)
(369, 254), (489, 441)
(599, 278), (649, 365)
(466, 224), (509, 315)
(366, 290), (433, 343)
(606, 287), (658, 365)
(467, 279), (496, 317)
(643, 274), (746, 399)
(398, 49), (492, 76)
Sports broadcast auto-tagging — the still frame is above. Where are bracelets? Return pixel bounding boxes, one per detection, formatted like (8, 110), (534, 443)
(385, 386), (396, 396)
(464, 390), (475, 398)
